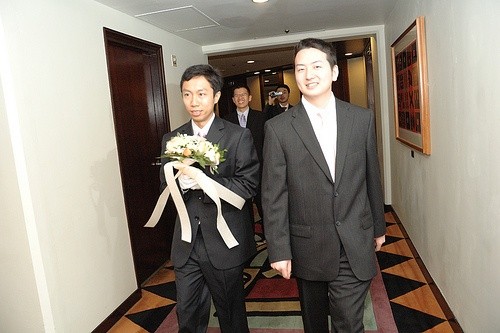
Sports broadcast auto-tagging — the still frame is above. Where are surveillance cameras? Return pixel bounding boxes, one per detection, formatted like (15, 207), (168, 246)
(285, 30), (289, 33)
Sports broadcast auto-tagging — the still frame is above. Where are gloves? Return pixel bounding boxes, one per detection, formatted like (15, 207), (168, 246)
(178, 173), (201, 190)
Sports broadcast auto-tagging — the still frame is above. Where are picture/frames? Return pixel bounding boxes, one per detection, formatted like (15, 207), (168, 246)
(390, 16), (432, 156)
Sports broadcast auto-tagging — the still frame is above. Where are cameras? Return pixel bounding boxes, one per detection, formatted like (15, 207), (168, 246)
(270, 91), (283, 97)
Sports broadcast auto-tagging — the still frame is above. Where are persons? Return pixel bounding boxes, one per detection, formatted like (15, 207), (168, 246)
(223, 83), (265, 238)
(263, 84), (295, 121)
(159, 64), (260, 333)
(260, 38), (386, 333)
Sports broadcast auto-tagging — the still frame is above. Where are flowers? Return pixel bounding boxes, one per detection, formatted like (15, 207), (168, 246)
(154, 131), (229, 175)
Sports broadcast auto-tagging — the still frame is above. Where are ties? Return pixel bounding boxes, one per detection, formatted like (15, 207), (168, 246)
(318, 109), (335, 182)
(281, 107), (287, 111)
(240, 114), (246, 128)
(198, 131), (205, 137)
